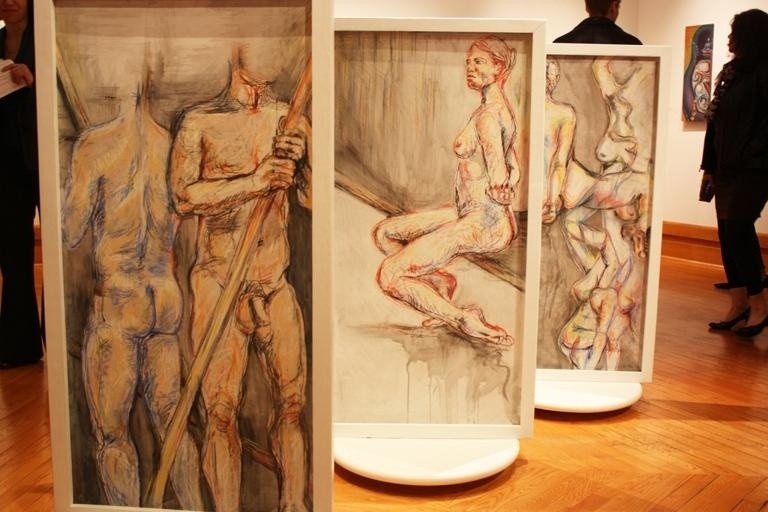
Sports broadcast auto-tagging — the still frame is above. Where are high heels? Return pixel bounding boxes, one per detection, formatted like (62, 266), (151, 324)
(736, 312), (768, 337)
(708, 306), (751, 330)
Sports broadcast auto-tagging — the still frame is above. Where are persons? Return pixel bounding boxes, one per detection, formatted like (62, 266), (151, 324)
(0, 0), (44, 370)
(371, 34), (521, 347)
(700, 9), (768, 338)
(59, 78), (204, 510)
(552, 0), (643, 44)
(542, 57), (653, 371)
(166, 43), (313, 512)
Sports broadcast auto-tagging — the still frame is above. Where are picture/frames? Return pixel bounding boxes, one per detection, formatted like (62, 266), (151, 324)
(33, 0), (335, 512)
(332, 17), (547, 439)
(537, 43), (672, 383)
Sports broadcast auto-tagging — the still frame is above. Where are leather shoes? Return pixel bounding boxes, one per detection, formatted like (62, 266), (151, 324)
(714, 275), (768, 290)
